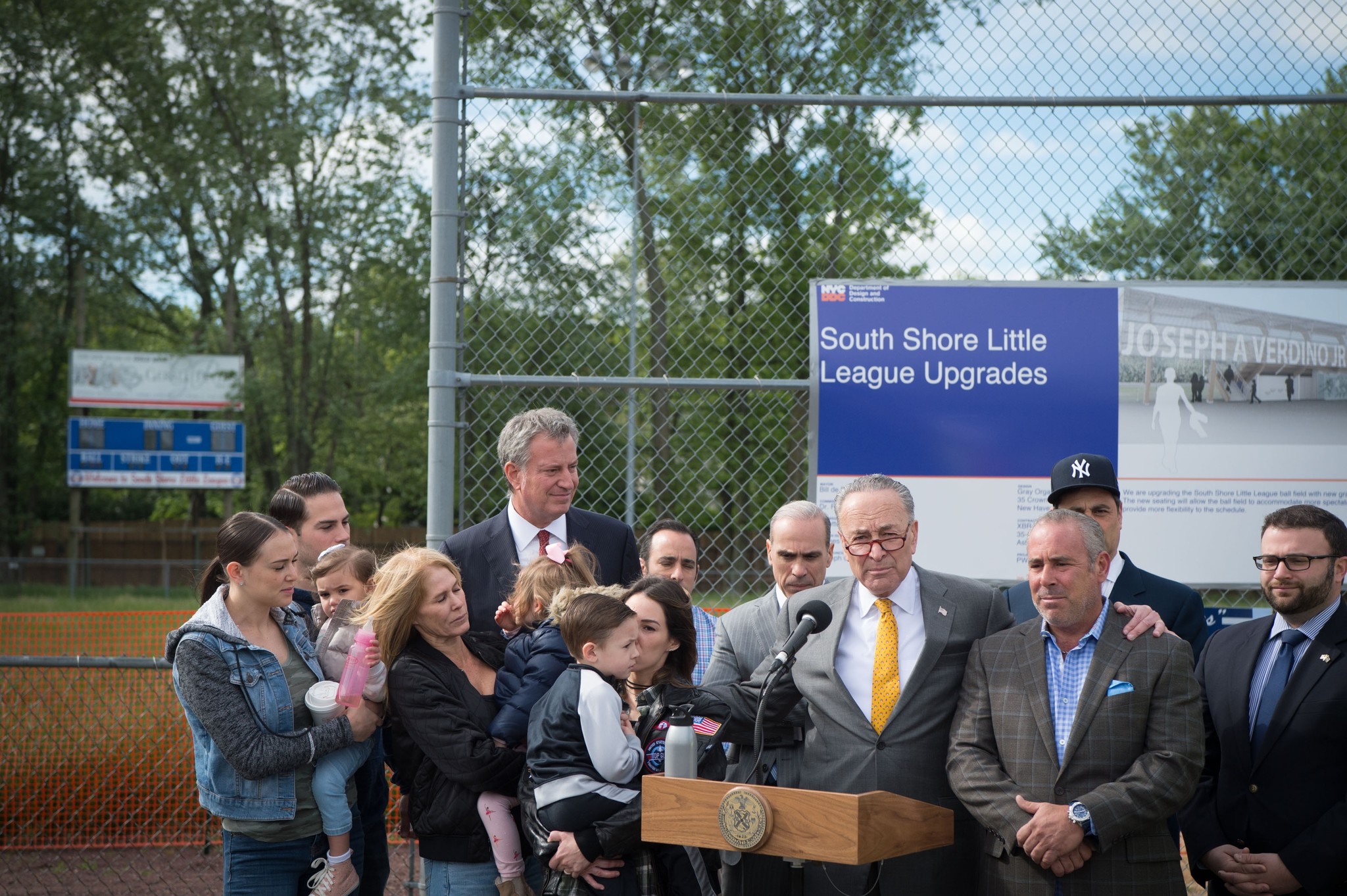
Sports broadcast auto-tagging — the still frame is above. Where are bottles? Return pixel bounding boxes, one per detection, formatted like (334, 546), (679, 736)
(664, 704), (698, 779)
(334, 615), (376, 709)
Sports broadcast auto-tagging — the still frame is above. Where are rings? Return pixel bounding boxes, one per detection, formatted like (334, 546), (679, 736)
(564, 869), (572, 875)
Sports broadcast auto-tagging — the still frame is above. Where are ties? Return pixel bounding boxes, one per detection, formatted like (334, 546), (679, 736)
(1250, 628), (1307, 769)
(536, 530), (551, 557)
(871, 598), (900, 736)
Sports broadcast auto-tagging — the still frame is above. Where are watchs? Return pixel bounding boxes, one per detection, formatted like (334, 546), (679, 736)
(1067, 801), (1092, 835)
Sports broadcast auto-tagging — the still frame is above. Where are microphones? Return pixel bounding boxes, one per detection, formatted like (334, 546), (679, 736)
(768, 600), (833, 673)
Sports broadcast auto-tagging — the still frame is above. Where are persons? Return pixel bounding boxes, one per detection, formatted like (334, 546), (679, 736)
(163, 511), (382, 895)
(1224, 365), (1234, 394)
(699, 500), (835, 894)
(946, 508), (1206, 896)
(307, 544), (388, 896)
(1182, 504), (1347, 896)
(1285, 374), (1294, 402)
(527, 594), (702, 896)
(1002, 452), (1207, 671)
(697, 474), (1182, 895)
(349, 547), (527, 895)
(518, 575), (731, 895)
(1250, 379), (1261, 404)
(1190, 372), (1205, 402)
(435, 408), (643, 653)
(640, 518), (731, 754)
(477, 544), (599, 896)
(270, 472), (350, 622)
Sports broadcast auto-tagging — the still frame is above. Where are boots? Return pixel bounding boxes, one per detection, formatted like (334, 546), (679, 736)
(494, 873), (536, 896)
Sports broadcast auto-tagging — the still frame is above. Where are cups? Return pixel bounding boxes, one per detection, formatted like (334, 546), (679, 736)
(305, 680), (345, 727)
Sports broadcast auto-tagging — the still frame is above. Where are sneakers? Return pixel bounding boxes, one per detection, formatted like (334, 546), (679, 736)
(308, 857), (360, 896)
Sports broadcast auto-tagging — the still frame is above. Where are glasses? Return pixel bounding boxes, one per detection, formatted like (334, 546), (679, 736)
(844, 524), (909, 556)
(1253, 555), (1337, 572)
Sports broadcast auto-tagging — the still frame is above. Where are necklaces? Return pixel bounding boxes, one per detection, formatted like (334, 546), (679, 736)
(624, 679), (655, 701)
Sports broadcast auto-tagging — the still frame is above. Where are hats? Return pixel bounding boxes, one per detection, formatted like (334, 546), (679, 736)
(1048, 453), (1121, 506)
